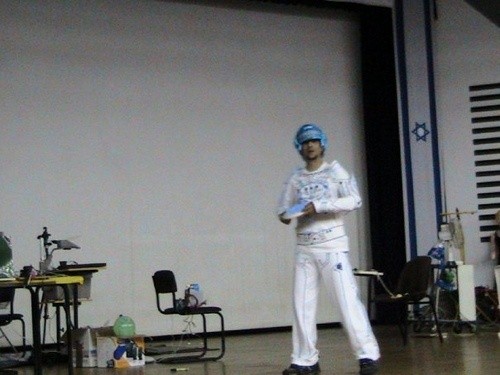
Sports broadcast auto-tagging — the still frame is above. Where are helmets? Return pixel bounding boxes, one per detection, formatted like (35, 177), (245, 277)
(293, 124), (328, 151)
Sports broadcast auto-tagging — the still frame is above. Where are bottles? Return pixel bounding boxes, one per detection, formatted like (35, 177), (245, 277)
(113, 316), (135, 339)
(82, 326), (96, 367)
(189, 284), (200, 307)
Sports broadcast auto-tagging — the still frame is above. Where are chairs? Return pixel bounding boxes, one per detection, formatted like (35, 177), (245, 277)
(152, 270), (226, 365)
(368, 255), (444, 346)
(0, 272), (27, 360)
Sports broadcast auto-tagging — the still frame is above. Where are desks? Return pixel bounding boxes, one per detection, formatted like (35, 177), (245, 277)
(352, 269), (384, 299)
(0, 276), (83, 375)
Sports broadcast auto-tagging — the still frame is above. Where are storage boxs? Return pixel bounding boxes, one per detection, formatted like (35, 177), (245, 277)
(97, 334), (146, 368)
(61, 325), (116, 368)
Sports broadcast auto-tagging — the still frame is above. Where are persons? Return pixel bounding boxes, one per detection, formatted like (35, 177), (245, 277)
(276, 124), (386, 375)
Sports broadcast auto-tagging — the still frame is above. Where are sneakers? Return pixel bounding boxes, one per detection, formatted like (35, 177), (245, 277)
(360, 359), (381, 375)
(282, 363), (320, 375)
